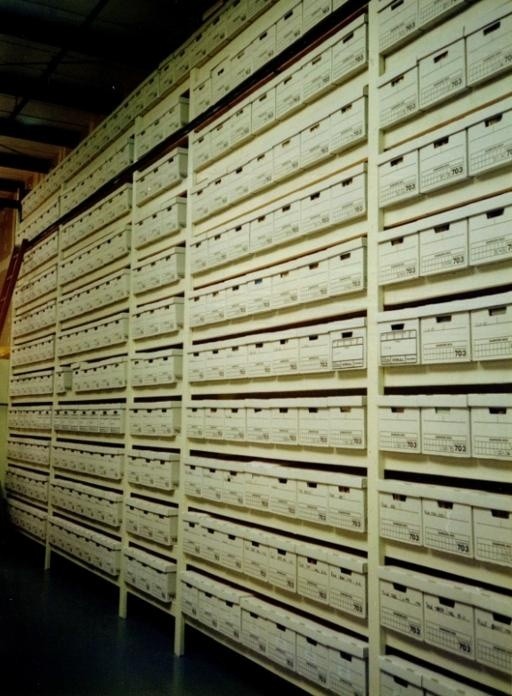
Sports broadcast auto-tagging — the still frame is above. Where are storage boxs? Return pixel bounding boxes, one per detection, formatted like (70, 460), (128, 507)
(0, 0), (512, 695)
